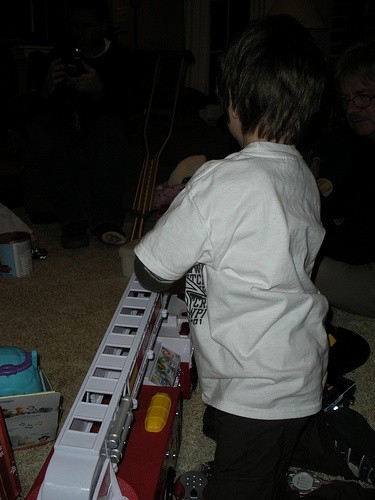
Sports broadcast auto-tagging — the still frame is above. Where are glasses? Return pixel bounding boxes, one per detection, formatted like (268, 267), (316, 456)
(339, 94), (374, 109)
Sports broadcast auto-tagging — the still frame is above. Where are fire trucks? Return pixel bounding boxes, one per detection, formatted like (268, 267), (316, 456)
(24, 270), (200, 499)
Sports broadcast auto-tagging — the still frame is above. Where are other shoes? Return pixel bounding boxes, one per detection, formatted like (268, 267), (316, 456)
(326, 480), (375, 500)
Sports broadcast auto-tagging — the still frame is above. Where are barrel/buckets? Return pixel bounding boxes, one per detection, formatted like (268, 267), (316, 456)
(0, 231), (33, 279)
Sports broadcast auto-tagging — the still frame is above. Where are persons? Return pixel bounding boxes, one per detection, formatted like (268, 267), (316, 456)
(301, 42), (375, 319)
(133, 11), (334, 500)
